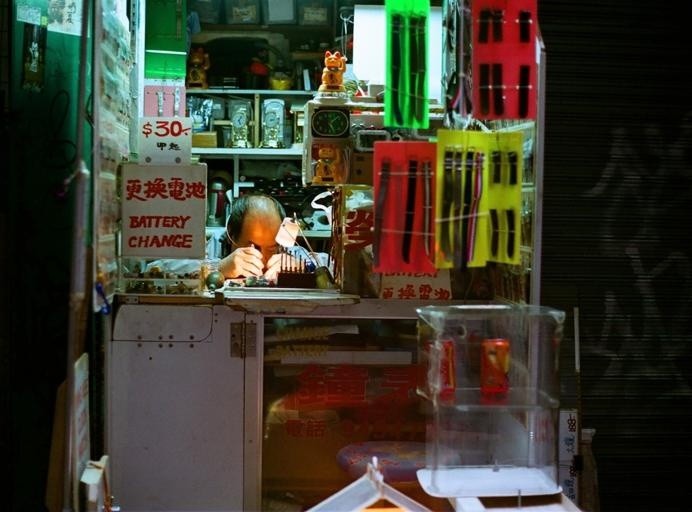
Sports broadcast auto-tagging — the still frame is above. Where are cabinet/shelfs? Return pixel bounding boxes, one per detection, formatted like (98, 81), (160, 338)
(183, 84), (342, 271)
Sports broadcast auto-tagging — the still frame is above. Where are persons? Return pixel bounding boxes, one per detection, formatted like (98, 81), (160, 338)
(147, 191), (332, 283)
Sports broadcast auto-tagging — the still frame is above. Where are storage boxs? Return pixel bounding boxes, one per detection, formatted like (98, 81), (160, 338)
(417, 305), (568, 497)
